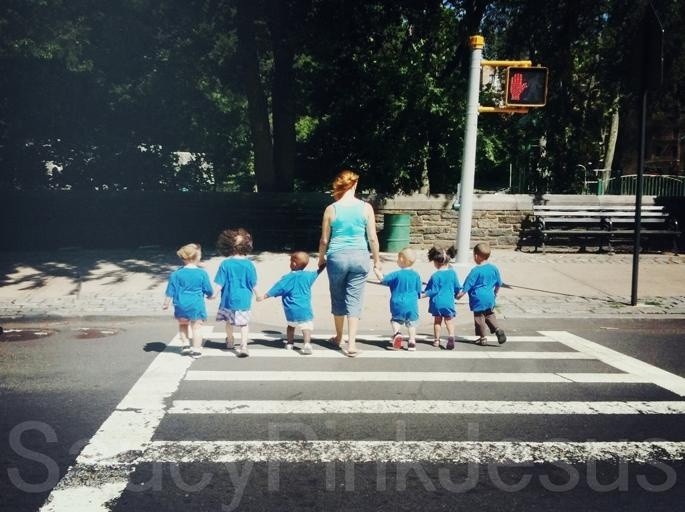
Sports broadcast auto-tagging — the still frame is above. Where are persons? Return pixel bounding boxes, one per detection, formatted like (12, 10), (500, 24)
(455, 242), (510, 345)
(207, 228), (262, 357)
(372, 248), (423, 350)
(417, 245), (462, 350)
(318, 170), (383, 357)
(163, 242), (214, 356)
(256, 252), (325, 355)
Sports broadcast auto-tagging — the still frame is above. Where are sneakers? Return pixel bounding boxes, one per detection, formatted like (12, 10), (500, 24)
(226, 334), (248, 357)
(433, 336), (455, 349)
(392, 331), (415, 350)
(475, 327), (506, 345)
(181, 343), (203, 358)
(286, 341), (312, 354)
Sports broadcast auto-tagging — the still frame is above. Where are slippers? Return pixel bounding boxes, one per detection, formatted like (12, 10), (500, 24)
(328, 337), (359, 357)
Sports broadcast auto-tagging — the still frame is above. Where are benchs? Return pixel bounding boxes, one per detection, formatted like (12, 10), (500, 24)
(533, 204), (684, 257)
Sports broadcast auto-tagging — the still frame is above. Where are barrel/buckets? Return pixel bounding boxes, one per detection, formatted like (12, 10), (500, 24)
(383, 213), (410, 252)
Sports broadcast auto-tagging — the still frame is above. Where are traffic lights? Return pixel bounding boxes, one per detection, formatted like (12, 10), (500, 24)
(505, 66), (549, 108)
